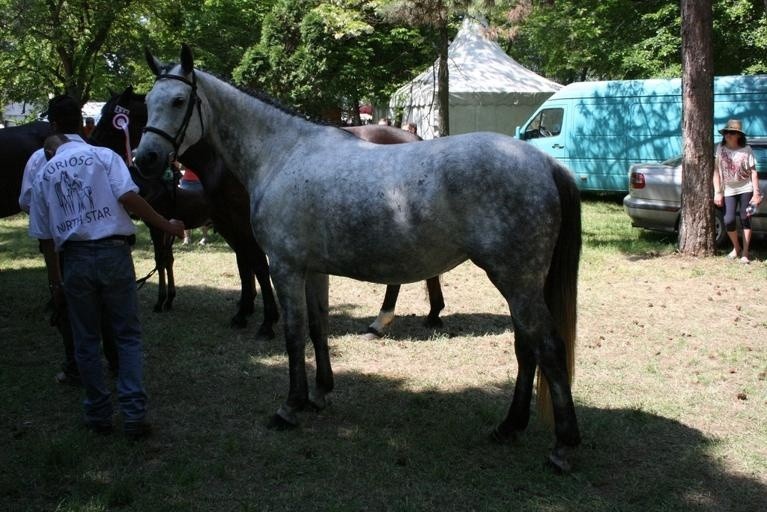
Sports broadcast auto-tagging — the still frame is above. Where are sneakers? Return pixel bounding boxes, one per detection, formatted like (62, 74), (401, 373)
(53, 369), (78, 386)
(728, 248), (740, 260)
(77, 412), (118, 437)
(739, 255), (753, 264)
(122, 418), (154, 439)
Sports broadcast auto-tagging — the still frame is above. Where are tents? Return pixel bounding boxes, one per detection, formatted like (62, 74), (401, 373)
(385, 7), (567, 145)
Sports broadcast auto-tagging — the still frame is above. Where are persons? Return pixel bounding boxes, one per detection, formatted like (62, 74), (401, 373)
(27, 131), (188, 438)
(82, 116), (97, 140)
(19, 95), (118, 386)
(712, 119), (762, 266)
(178, 168), (209, 249)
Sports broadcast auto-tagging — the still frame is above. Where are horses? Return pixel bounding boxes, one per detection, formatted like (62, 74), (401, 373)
(122, 160), (210, 313)
(0, 120), (52, 253)
(88, 85), (445, 343)
(134, 42), (582, 475)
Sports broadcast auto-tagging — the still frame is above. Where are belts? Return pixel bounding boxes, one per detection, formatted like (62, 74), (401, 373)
(100, 233), (136, 248)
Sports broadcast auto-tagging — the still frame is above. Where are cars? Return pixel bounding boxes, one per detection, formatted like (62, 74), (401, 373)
(624, 138), (767, 245)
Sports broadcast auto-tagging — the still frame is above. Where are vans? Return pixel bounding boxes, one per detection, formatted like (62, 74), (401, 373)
(512, 74), (767, 199)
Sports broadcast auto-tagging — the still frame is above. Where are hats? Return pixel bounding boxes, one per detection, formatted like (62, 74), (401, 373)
(718, 118), (749, 137)
(38, 94), (81, 118)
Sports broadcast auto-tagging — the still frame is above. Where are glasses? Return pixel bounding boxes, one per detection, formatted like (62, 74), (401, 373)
(723, 130), (740, 136)
